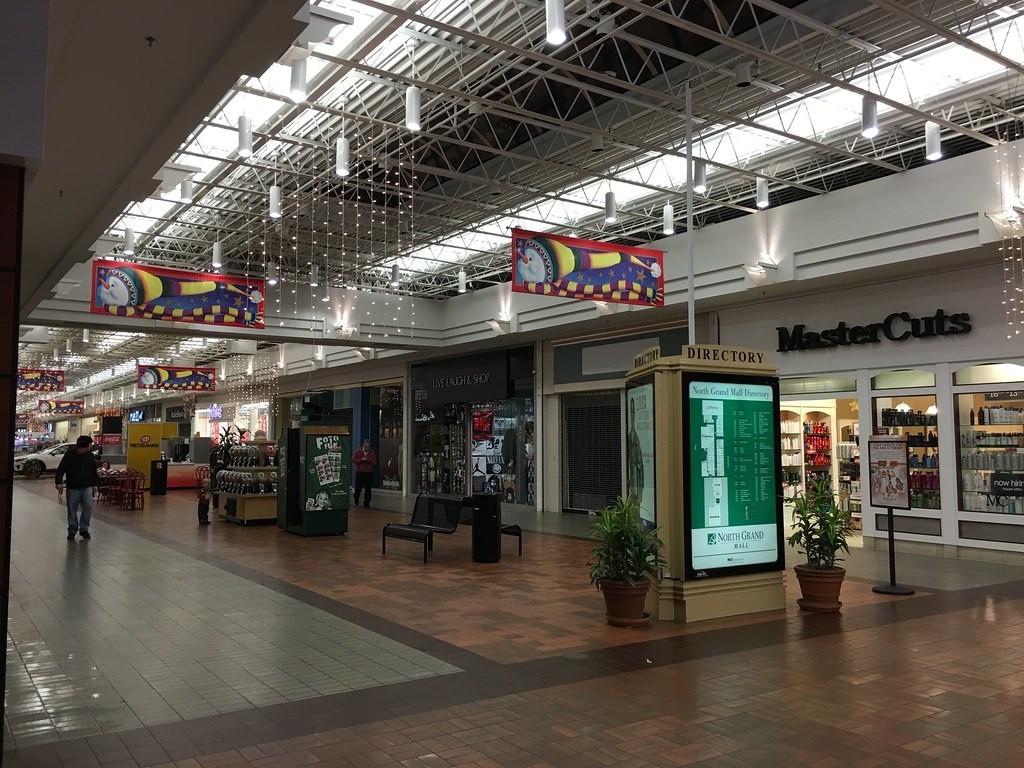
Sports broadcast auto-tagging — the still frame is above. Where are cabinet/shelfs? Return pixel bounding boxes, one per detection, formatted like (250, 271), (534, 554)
(872, 395), (941, 510)
(953, 392), (1024, 517)
(779, 410), (860, 510)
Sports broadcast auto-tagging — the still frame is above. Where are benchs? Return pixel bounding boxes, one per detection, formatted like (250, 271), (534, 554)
(382, 493), (522, 562)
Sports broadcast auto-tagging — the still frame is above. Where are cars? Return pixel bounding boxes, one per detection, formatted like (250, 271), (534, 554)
(14, 443), (94, 478)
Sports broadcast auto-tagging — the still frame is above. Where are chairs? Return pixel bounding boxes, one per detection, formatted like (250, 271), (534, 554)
(92, 466), (149, 510)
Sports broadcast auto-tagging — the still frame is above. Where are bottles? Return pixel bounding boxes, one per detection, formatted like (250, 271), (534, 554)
(908, 454), (939, 468)
(962, 473), (987, 492)
(781, 437), (798, 450)
(804, 420), (829, 433)
(882, 408), (937, 426)
(964, 493), (987, 510)
(910, 489), (939, 508)
(970, 405), (1024, 425)
(928, 431), (933, 440)
(853, 518), (862, 531)
(780, 420), (799, 433)
(836, 442), (857, 458)
(822, 472), (824, 479)
(910, 471), (939, 489)
(962, 450), (1024, 470)
(1008, 500), (1014, 513)
(807, 470), (810, 480)
(805, 455), (810, 462)
(960, 433), (1024, 448)
(782, 469), (802, 498)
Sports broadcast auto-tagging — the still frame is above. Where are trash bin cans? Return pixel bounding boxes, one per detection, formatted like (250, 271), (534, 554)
(472, 492), (501, 563)
(150, 460), (167, 495)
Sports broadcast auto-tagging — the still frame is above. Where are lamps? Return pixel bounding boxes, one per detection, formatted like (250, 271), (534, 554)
(268, 259), (278, 285)
(663, 199), (675, 235)
(545, 0), (566, 45)
(269, 151), (283, 218)
(53, 328), (89, 360)
(861, 95), (878, 139)
(605, 181), (616, 224)
(391, 264), (400, 288)
(406, 40), (421, 132)
(755, 168), (770, 209)
(212, 214), (223, 268)
(238, 108), (254, 157)
(336, 95), (350, 177)
(925, 121), (942, 161)
(693, 132), (707, 194)
(125, 229), (134, 257)
(310, 263), (319, 288)
(290, 59), (307, 102)
(181, 180), (193, 204)
(458, 267), (467, 293)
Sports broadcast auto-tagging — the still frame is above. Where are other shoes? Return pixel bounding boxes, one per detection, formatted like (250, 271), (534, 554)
(67, 533), (75, 540)
(80, 530), (90, 539)
(355, 497), (359, 505)
(200, 519), (211, 525)
(364, 504), (370, 509)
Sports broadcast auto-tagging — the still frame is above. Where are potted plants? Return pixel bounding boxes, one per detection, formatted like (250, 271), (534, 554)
(585, 491), (670, 628)
(777, 474), (857, 613)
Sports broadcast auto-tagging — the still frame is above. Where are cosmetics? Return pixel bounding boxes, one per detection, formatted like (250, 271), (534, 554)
(962, 405), (1024, 514)
(780, 420), (831, 514)
(882, 408), (939, 509)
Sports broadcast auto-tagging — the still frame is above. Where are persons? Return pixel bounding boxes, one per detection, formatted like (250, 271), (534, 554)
(306, 489), (331, 511)
(102, 461), (110, 469)
(198, 479), (222, 524)
(352, 439), (376, 508)
(55, 435), (98, 540)
(872, 470), (904, 499)
(627, 398), (644, 525)
